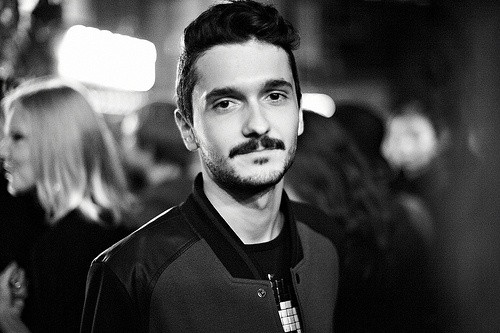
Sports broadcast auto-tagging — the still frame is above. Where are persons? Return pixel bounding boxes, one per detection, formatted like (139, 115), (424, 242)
(81, 0), (348, 333)
(0, 0), (461, 333)
(0, 85), (139, 333)
